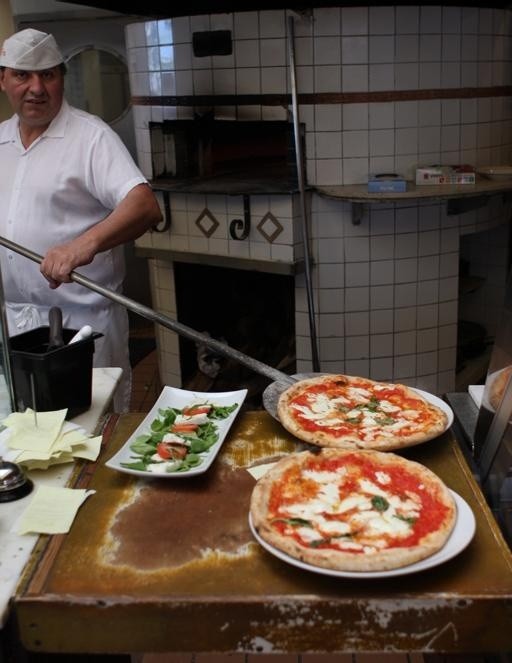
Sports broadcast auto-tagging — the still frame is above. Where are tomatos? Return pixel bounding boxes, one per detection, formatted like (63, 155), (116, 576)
(158, 442), (188, 459)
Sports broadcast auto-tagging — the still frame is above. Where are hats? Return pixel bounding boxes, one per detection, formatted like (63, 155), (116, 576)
(0, 29), (64, 71)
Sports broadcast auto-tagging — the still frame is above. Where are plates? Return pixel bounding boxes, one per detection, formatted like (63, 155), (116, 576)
(396, 387), (456, 434)
(246, 489), (478, 580)
(106, 385), (250, 477)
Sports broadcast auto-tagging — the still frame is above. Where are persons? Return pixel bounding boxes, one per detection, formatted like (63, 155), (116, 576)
(1, 29), (162, 413)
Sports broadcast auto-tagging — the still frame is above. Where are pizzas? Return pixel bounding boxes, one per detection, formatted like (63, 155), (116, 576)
(480, 364), (511, 422)
(247, 448), (476, 577)
(277, 375), (454, 450)
